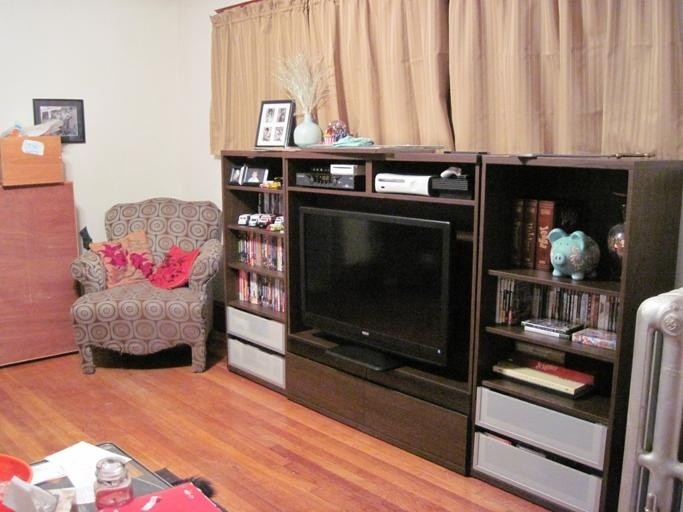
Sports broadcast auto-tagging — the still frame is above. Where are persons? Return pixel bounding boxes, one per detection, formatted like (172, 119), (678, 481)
(261, 107), (285, 141)
(230, 168), (240, 182)
(43, 106), (76, 138)
(247, 171), (259, 182)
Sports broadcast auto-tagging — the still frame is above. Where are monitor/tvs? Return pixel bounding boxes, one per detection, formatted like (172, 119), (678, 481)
(296, 207), (451, 372)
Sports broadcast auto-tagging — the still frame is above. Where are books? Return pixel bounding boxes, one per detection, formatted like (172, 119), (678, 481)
(535, 200), (583, 272)
(522, 200), (537, 270)
(494, 274), (622, 403)
(101, 480), (223, 512)
(510, 195), (521, 265)
(237, 190), (287, 313)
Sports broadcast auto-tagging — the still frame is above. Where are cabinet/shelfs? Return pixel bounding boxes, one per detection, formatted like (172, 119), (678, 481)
(281, 147), (481, 480)
(219, 148), (287, 396)
(471, 153), (679, 511)
(1, 183), (89, 368)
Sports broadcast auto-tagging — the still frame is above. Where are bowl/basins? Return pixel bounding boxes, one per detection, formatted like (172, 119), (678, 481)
(0, 453), (35, 512)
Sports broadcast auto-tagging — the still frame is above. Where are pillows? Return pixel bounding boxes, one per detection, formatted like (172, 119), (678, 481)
(88, 230), (154, 291)
(155, 244), (198, 294)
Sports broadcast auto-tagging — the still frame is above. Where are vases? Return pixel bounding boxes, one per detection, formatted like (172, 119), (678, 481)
(292, 114), (323, 148)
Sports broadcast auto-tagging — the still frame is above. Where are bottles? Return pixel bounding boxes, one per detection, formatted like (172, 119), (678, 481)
(93, 456), (132, 511)
(311, 167), (332, 184)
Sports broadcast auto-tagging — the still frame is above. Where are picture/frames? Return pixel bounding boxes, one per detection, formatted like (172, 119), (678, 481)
(255, 100), (293, 149)
(33, 98), (85, 144)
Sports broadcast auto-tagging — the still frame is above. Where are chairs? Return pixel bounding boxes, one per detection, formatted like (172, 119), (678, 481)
(70, 197), (219, 374)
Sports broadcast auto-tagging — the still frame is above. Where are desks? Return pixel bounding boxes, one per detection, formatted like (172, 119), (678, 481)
(1, 442), (221, 512)
